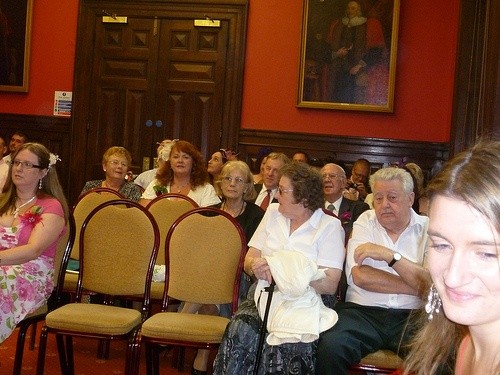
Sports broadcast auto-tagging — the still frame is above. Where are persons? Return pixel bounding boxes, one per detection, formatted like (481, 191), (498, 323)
(139, 140), (221, 207)
(314, 168), (434, 375)
(320, 163), (370, 230)
(345, 158), (373, 202)
(81, 147), (142, 208)
(3, 131), (26, 163)
(134, 139), (172, 190)
(207, 149), (228, 196)
(0, 136), (9, 194)
(291, 151), (310, 165)
(397, 142), (500, 375)
(402, 163), (430, 216)
(0, 143), (69, 344)
(244, 149), (290, 211)
(212, 165), (346, 375)
(160, 161), (265, 375)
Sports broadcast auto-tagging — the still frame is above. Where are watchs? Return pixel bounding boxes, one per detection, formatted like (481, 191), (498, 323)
(388, 253), (401, 267)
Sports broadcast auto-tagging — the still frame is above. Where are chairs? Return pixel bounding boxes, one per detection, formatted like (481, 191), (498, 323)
(9, 188), (408, 375)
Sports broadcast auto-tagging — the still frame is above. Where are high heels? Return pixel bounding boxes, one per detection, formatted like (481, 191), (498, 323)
(190, 352), (208, 375)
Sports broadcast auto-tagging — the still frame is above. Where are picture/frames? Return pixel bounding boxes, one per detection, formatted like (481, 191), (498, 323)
(0, 0), (34, 93)
(296, 0), (400, 113)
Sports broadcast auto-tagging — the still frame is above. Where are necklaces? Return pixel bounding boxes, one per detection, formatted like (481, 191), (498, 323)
(10, 196), (36, 217)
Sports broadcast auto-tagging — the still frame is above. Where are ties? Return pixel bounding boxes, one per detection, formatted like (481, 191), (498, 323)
(260, 189), (272, 210)
(327, 204), (335, 212)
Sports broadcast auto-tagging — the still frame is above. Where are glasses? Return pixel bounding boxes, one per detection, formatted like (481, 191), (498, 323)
(354, 172), (367, 181)
(108, 160), (128, 167)
(277, 186), (294, 196)
(11, 159), (41, 170)
(223, 177), (247, 185)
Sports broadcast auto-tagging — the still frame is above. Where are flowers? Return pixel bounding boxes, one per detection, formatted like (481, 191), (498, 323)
(341, 211), (352, 222)
